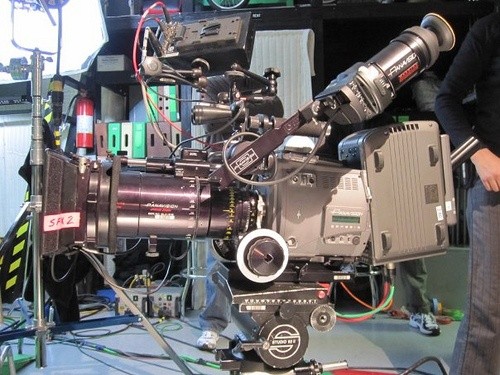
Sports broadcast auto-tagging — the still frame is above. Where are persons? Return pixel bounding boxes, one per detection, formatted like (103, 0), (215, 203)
(400, 259), (440, 336)
(436, 12), (500, 375)
(197, 75), (232, 350)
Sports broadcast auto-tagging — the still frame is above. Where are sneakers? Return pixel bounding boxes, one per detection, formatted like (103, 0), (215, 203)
(196, 328), (219, 350)
(409, 313), (442, 336)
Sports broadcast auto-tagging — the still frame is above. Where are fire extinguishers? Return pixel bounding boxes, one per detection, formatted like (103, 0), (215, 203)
(63, 87), (93, 148)
